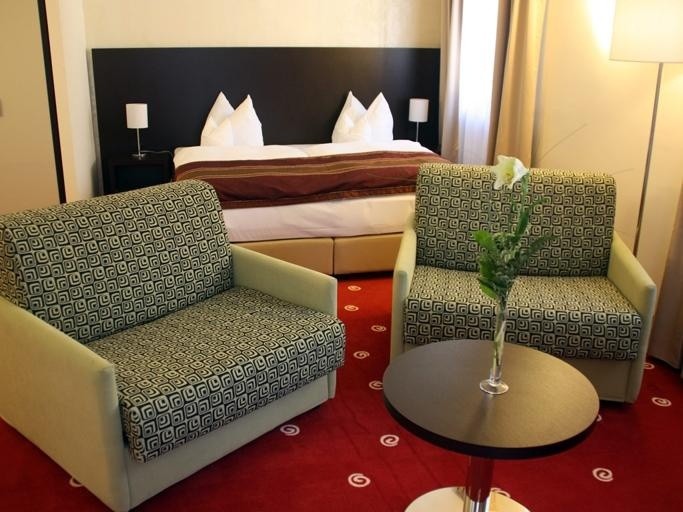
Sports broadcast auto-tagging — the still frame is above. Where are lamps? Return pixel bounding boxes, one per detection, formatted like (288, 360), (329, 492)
(126, 103), (169, 159)
(609, 0), (682, 257)
(409, 99), (429, 142)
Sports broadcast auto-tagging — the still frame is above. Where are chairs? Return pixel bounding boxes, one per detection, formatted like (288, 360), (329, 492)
(389, 163), (654, 404)
(0, 179), (347, 512)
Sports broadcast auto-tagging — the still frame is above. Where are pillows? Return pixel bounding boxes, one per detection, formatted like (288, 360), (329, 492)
(211, 95), (265, 146)
(332, 91), (366, 143)
(200, 91), (236, 146)
(341, 91), (394, 142)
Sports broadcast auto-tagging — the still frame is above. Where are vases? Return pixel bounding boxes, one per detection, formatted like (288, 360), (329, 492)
(480, 300), (508, 398)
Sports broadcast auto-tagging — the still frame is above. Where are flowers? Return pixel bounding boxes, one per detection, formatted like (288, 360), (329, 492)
(477, 154), (557, 363)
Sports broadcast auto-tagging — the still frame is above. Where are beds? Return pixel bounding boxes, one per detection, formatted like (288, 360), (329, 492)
(172, 139), (454, 274)
